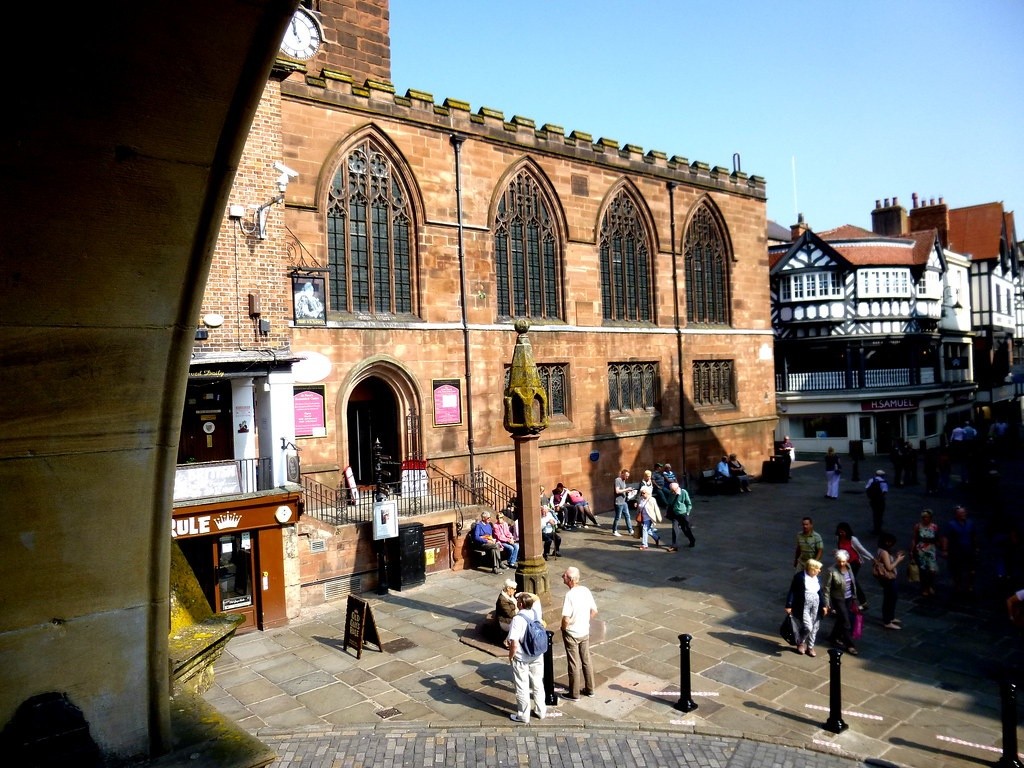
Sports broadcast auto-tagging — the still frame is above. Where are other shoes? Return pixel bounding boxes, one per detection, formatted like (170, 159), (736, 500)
(797, 643), (805, 654)
(561, 693), (581, 701)
(808, 647), (816, 657)
(503, 641), (510, 650)
(612, 530), (622, 536)
(494, 523), (602, 574)
(640, 546), (648, 550)
(842, 618), (903, 655)
(656, 537), (660, 547)
(689, 539), (696, 547)
(629, 529), (635, 535)
(580, 689), (594, 697)
(510, 714), (523, 721)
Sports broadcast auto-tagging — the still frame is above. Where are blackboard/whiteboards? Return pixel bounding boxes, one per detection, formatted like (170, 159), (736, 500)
(343, 594), (384, 652)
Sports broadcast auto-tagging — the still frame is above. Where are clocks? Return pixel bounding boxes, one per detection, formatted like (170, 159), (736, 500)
(278, 10), (320, 60)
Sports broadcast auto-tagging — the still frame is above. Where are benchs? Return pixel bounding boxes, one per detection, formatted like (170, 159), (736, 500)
(468, 526), (545, 570)
(701, 469), (754, 496)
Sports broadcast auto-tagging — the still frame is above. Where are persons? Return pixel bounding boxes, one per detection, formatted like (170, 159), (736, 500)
(503, 592), (547, 723)
(890, 441), (921, 487)
(950, 421), (977, 454)
(715, 454), (753, 493)
(475, 483), (601, 575)
(865, 469), (888, 535)
(779, 437), (793, 478)
(496, 579), (519, 649)
(823, 447), (842, 499)
(909, 509), (949, 596)
(612, 463), (696, 552)
(1007, 589), (1024, 625)
(779, 517), (908, 657)
(940, 504), (978, 592)
(560, 567), (598, 701)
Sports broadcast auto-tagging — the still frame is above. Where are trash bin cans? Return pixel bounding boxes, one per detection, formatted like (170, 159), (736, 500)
(769, 455), (786, 483)
(385, 522), (427, 592)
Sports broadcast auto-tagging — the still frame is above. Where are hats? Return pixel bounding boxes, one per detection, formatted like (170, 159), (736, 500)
(654, 463), (663, 468)
(876, 470), (885, 475)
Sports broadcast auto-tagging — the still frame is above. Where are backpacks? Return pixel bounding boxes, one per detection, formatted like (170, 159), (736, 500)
(517, 608), (547, 656)
(866, 476), (887, 500)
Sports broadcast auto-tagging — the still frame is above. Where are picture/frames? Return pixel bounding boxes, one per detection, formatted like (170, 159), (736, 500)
(292, 274), (327, 326)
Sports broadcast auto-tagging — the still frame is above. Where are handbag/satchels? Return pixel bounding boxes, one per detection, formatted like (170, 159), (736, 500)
(636, 512), (642, 522)
(907, 558), (919, 582)
(780, 613), (802, 645)
(853, 603), (863, 640)
(835, 464), (842, 475)
(872, 550), (896, 579)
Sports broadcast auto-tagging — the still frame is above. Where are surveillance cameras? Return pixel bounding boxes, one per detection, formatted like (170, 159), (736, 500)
(273, 162), (299, 178)
